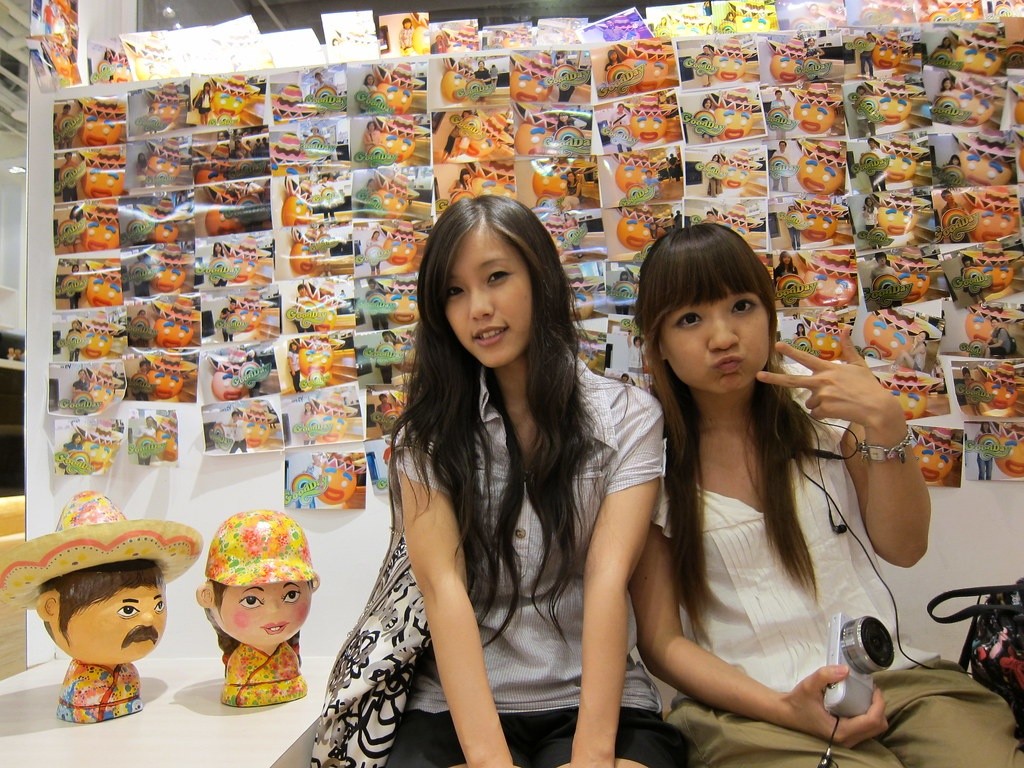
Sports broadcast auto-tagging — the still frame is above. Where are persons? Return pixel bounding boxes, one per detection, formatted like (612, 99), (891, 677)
(769, 2), (1024, 481)
(627, 221), (1024, 768)
(55, 69), (284, 476)
(193, 510), (321, 708)
(380, 192), (705, 768)
(509, 1), (775, 395)
(0, 493), (201, 723)
(271, 11), (509, 443)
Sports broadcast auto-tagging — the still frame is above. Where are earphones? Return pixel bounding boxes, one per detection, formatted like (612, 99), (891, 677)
(829, 509), (847, 533)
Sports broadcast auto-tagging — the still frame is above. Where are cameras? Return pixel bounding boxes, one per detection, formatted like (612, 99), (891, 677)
(824, 612), (894, 718)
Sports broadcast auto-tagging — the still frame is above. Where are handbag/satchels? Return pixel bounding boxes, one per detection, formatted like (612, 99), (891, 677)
(927, 577), (1024, 731)
(311, 437), (463, 768)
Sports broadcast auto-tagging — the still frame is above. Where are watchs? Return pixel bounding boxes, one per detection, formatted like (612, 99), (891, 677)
(856, 425), (915, 465)
